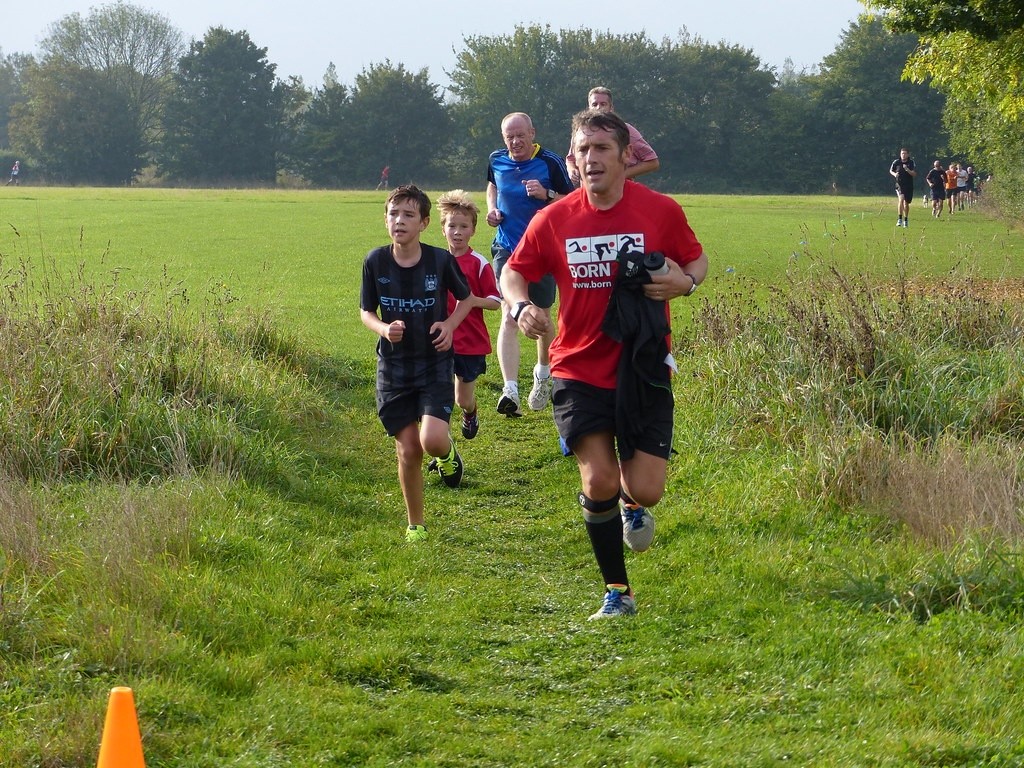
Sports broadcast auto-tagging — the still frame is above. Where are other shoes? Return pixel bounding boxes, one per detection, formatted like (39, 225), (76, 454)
(936, 214), (939, 219)
(904, 221), (908, 228)
(932, 209), (936, 215)
(896, 219), (902, 226)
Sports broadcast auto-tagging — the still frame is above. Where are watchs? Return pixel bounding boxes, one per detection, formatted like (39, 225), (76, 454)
(510, 300), (535, 323)
(545, 187), (555, 204)
(684, 272), (696, 297)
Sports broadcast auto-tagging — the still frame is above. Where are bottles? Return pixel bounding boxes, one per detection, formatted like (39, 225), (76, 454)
(643, 252), (668, 280)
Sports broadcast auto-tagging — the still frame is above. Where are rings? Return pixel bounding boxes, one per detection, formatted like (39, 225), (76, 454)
(656, 290), (659, 296)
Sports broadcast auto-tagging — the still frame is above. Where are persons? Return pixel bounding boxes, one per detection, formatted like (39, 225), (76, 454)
(359, 185), (473, 547)
(376, 165), (389, 190)
(926, 160), (992, 218)
(499, 110), (709, 624)
(437, 190), (499, 440)
(889, 148), (917, 226)
(485, 112), (576, 417)
(565, 86), (660, 188)
(5, 160), (21, 186)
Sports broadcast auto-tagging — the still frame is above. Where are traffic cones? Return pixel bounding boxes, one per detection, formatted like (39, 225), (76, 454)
(96, 686), (146, 767)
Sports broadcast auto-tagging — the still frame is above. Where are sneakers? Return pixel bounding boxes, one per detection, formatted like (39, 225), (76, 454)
(404, 525), (430, 544)
(462, 409), (479, 439)
(437, 439), (464, 488)
(587, 584), (635, 621)
(497, 387), (522, 418)
(619, 497), (654, 553)
(428, 459), (438, 471)
(527, 364), (551, 412)
